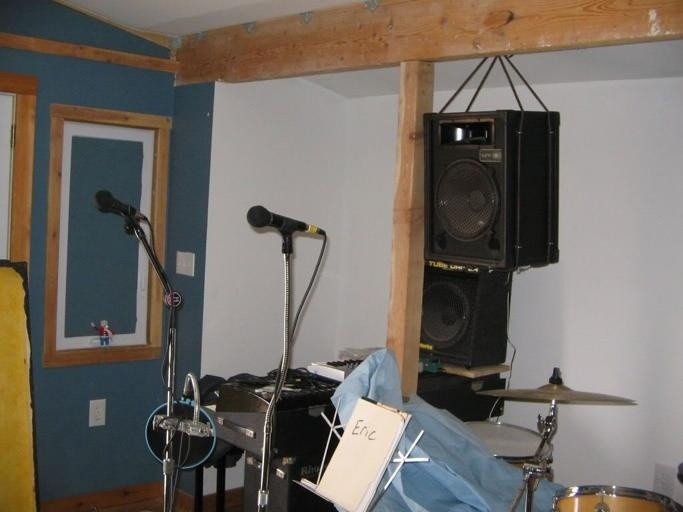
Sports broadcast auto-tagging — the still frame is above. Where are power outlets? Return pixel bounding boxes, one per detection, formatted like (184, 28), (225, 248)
(89, 399), (106, 427)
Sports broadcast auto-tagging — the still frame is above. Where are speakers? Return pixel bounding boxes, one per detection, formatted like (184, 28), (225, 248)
(419, 260), (508, 368)
(423, 109), (560, 270)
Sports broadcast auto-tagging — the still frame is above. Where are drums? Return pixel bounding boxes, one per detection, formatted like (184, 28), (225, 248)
(463, 421), (553, 475)
(553, 485), (682, 512)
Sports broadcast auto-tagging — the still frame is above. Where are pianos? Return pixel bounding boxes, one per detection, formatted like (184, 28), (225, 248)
(308, 358), (366, 382)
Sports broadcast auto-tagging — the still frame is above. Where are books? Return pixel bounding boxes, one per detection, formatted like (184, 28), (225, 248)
(314, 397), (412, 511)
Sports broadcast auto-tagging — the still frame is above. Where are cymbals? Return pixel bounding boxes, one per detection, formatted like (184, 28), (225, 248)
(478, 384), (635, 405)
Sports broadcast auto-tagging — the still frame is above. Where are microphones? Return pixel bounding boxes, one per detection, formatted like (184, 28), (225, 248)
(247, 205), (326, 234)
(93, 190), (149, 220)
